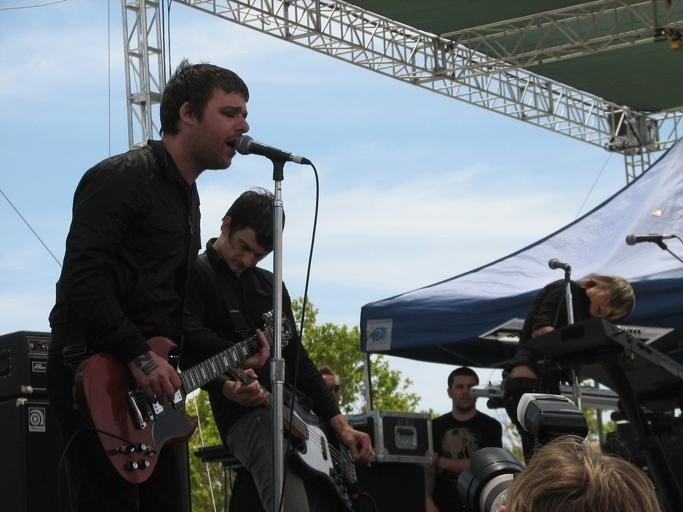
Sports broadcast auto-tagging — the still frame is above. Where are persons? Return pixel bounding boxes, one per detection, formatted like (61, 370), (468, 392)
(504, 436), (663, 512)
(182, 188), (375, 511)
(48, 62), (272, 512)
(318, 364), (340, 405)
(502, 275), (634, 469)
(423, 367), (502, 512)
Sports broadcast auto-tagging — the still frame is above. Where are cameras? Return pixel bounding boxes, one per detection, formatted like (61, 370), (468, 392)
(457, 393), (587, 512)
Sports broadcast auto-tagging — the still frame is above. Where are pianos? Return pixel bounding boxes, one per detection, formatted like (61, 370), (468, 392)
(525, 318), (682, 415)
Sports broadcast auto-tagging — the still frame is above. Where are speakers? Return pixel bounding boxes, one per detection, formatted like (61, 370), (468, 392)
(0, 397), (191, 511)
(350, 462), (426, 512)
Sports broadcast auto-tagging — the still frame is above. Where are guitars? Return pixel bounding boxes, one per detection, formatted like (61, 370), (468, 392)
(223, 366), (362, 511)
(73, 309), (293, 487)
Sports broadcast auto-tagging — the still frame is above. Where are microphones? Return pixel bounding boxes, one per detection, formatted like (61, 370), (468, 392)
(548, 257), (572, 271)
(625, 233), (674, 246)
(235, 135), (311, 165)
